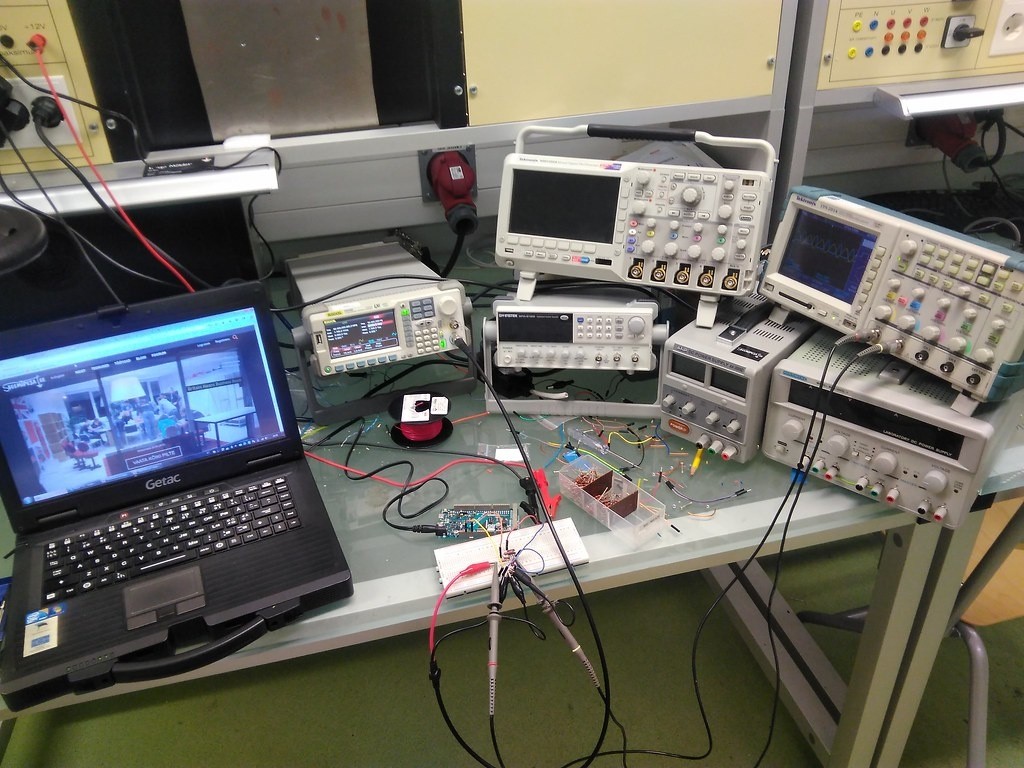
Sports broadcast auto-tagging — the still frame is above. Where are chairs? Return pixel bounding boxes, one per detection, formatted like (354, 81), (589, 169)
(795, 495), (1024, 768)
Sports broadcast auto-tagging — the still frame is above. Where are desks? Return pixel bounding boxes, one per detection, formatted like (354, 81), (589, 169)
(726, 187), (1024, 768)
(0, 244), (942, 768)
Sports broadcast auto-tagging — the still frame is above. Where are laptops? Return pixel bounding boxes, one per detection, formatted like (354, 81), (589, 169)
(0, 282), (354, 713)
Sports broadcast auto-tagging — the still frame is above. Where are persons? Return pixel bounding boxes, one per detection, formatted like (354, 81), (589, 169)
(75, 391), (182, 443)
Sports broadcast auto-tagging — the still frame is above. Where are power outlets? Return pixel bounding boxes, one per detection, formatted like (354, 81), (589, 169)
(905, 110), (988, 148)
(989, 0), (1024, 57)
(0, 75), (83, 151)
(417, 144), (478, 204)
